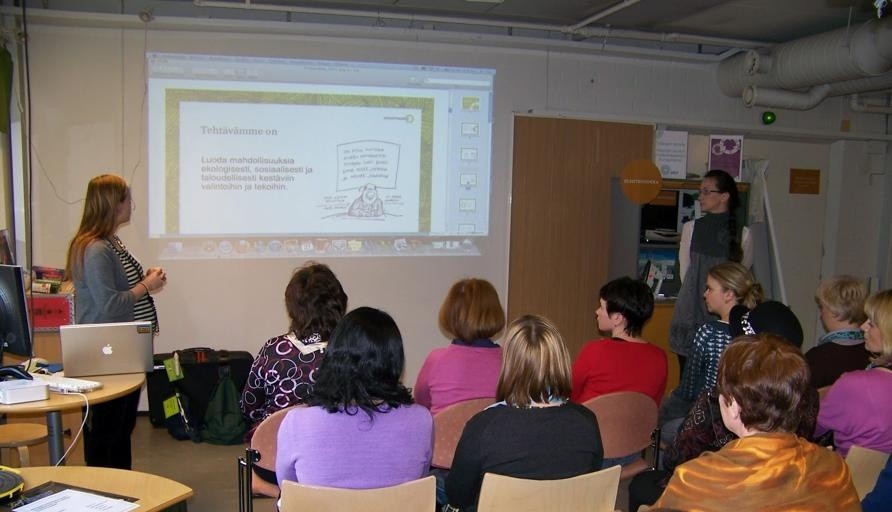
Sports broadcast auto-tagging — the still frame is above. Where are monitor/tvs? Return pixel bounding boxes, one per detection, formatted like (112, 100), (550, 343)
(0, 265), (33, 380)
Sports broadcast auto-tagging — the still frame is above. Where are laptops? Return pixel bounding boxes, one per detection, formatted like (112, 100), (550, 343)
(59, 319), (154, 378)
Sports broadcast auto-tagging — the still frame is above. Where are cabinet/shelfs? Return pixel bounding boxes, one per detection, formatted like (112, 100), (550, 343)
(614, 176), (751, 398)
(0, 326), (90, 468)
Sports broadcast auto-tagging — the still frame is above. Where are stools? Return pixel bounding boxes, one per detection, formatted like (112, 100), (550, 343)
(0, 423), (49, 468)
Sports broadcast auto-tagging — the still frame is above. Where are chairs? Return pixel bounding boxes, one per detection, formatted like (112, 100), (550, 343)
(238, 391), (662, 511)
(842, 445), (891, 501)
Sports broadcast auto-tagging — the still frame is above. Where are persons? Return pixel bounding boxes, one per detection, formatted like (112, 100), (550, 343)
(666, 169), (755, 384)
(61, 174), (167, 471)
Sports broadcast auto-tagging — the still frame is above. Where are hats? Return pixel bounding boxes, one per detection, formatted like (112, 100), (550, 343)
(726, 297), (806, 350)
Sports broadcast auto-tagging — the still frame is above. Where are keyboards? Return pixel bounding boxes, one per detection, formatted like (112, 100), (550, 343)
(49, 375), (102, 392)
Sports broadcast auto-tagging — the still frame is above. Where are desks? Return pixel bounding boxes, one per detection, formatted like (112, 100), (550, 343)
(0, 368), (147, 469)
(0, 462), (194, 512)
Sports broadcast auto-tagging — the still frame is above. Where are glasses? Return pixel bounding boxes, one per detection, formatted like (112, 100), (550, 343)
(699, 188), (721, 195)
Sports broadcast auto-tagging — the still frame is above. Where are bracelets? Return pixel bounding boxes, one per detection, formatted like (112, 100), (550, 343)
(138, 281), (149, 294)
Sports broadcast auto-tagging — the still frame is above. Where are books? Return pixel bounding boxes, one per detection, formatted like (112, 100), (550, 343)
(10, 480), (140, 512)
(31, 265), (65, 293)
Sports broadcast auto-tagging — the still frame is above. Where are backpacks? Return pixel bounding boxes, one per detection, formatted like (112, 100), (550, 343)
(197, 377), (249, 446)
